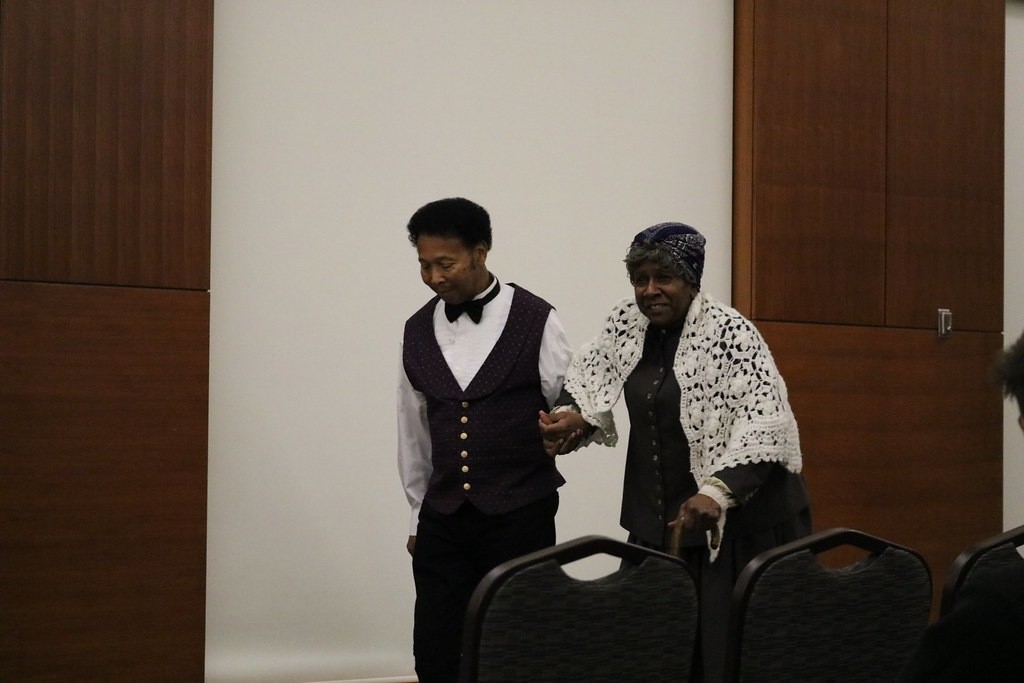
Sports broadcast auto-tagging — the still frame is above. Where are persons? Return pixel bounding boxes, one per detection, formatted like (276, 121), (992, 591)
(535, 221), (812, 579)
(397, 196), (580, 683)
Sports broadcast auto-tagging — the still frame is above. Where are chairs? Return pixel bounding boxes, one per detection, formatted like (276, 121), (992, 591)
(724, 527), (933, 683)
(939, 524), (1024, 618)
(460, 535), (703, 683)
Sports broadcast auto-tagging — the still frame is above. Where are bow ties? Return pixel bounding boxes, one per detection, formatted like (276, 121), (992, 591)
(444, 276), (500, 324)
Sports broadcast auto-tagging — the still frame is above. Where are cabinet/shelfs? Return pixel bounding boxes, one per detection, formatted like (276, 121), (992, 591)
(0, 1), (214, 683)
(732, 0), (1004, 621)
